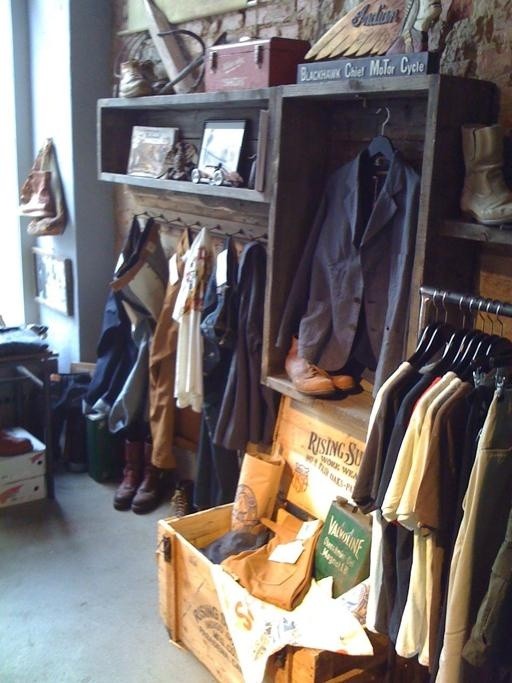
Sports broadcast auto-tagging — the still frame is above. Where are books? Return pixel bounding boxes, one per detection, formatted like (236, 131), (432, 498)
(127, 124), (180, 181)
(312, 495), (373, 599)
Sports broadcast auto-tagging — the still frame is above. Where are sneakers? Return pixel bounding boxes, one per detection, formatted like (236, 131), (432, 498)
(330, 374), (357, 394)
(168, 478), (194, 518)
(118, 60), (152, 98)
(138, 60), (173, 95)
(284, 334), (337, 397)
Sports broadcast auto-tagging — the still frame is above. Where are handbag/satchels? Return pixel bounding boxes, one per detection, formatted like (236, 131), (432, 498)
(19, 140), (56, 217)
(27, 140), (66, 236)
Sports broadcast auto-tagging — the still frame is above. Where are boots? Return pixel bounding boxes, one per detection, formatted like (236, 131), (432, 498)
(115, 430), (147, 510)
(458, 121), (512, 226)
(131, 433), (175, 514)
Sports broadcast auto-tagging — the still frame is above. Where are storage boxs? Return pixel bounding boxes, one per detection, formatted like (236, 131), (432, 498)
(1, 473), (48, 508)
(0, 425), (47, 484)
(203, 34), (314, 90)
(155, 502), (387, 683)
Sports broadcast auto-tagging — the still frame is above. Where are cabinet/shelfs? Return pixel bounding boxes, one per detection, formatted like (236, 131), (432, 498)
(94, 84), (272, 202)
(258, 73), (512, 422)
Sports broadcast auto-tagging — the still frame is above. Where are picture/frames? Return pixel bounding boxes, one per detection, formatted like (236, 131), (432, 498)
(30, 246), (72, 318)
(195, 118), (249, 186)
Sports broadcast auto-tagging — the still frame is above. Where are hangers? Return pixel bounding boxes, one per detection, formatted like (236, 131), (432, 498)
(414, 288), (511, 387)
(359, 103), (395, 174)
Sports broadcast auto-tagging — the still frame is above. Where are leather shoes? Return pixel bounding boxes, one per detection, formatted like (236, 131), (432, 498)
(0, 428), (32, 457)
